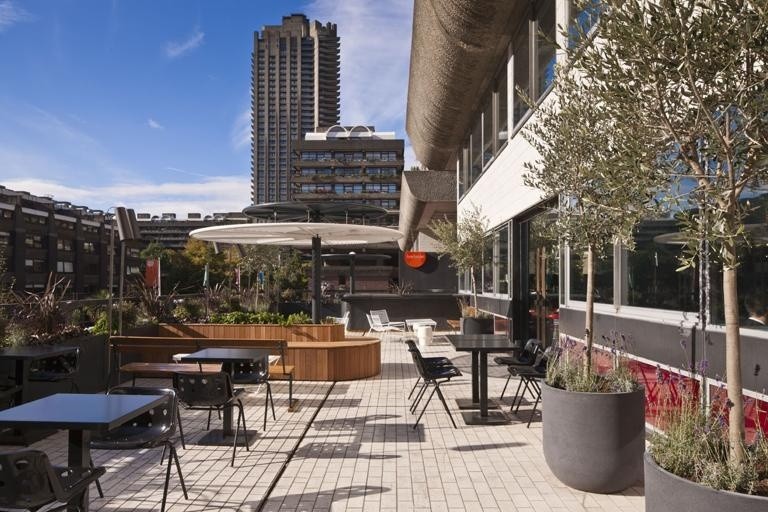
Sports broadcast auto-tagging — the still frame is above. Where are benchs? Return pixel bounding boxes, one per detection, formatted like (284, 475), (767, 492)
(109, 338), (302, 412)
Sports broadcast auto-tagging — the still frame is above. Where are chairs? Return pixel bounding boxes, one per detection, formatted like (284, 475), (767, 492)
(365, 314), (403, 343)
(494, 338), (543, 401)
(83, 387), (189, 511)
(404, 339), (453, 413)
(447, 305), (478, 330)
(410, 349), (462, 430)
(508, 346), (563, 429)
(371, 309), (405, 341)
(172, 370), (249, 468)
(330, 311), (350, 336)
(0, 449), (106, 511)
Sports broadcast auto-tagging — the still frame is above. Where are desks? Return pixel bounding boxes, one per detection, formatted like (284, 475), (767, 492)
(453, 333), (507, 426)
(406, 319), (437, 337)
(0, 392), (170, 512)
(183, 348), (275, 432)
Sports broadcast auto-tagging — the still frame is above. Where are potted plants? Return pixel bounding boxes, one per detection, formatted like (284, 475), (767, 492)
(645, 1), (768, 511)
(514, 0), (671, 494)
(427, 201), (495, 333)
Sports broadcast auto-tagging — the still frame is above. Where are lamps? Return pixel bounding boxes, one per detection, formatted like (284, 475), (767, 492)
(117, 209), (143, 385)
(106, 206), (134, 383)
